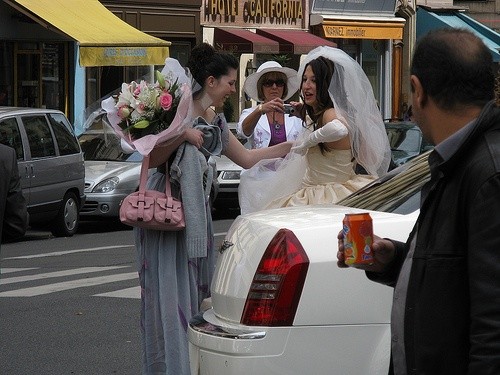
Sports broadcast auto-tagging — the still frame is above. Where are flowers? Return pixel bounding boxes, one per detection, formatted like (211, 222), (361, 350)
(114, 69), (184, 140)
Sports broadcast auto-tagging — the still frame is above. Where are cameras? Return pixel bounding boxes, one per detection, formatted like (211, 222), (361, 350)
(282, 104), (296, 114)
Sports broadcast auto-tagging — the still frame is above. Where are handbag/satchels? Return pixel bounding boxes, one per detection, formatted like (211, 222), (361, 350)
(119, 153), (186, 231)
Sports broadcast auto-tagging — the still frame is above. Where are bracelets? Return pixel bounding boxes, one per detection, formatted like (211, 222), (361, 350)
(259, 105), (265, 115)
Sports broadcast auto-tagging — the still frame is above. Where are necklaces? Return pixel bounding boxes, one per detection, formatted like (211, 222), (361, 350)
(269, 113), (280, 129)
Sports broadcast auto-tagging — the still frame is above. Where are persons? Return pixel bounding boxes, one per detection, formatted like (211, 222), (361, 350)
(236, 61), (310, 180)
(282, 46), (391, 207)
(337, 30), (500, 375)
(133, 43), (301, 375)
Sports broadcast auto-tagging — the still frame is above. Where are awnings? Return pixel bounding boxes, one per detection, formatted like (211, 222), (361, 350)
(256, 29), (337, 55)
(416, 8), (500, 62)
(3, 0), (171, 67)
(214, 27), (279, 54)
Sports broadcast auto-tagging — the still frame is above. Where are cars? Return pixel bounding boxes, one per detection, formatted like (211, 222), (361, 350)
(352, 118), (435, 176)
(202, 122), (243, 215)
(76, 130), (147, 229)
(185, 148), (434, 375)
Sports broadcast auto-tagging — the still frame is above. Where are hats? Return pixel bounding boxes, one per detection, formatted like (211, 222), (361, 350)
(242, 60), (303, 102)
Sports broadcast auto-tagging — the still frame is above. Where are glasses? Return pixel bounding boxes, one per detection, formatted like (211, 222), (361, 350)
(262, 79), (285, 87)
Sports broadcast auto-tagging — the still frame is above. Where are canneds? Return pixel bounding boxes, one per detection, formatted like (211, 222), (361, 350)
(342, 212), (374, 267)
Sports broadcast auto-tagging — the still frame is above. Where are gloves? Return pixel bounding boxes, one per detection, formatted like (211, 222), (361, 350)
(289, 119), (349, 156)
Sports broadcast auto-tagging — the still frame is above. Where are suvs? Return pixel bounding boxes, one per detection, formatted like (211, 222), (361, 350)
(0, 106), (87, 236)
